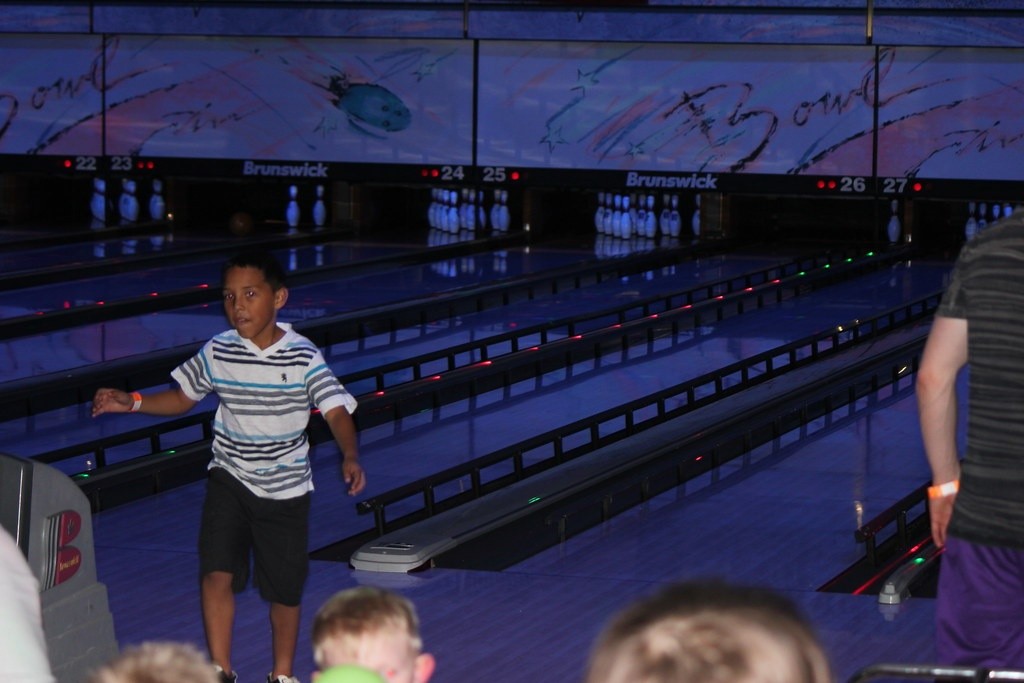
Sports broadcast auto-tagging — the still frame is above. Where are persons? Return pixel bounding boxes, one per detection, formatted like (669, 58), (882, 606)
(309, 584), (435, 683)
(93, 639), (220, 683)
(915, 211), (1024, 672)
(589, 578), (833, 683)
(0, 525), (57, 683)
(90, 251), (366, 683)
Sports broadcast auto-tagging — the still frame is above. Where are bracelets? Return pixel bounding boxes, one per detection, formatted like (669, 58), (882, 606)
(927, 480), (960, 499)
(129, 391), (142, 412)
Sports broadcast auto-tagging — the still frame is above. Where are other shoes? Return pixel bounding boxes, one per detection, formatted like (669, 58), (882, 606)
(267, 672), (300, 683)
(213, 664), (238, 683)
